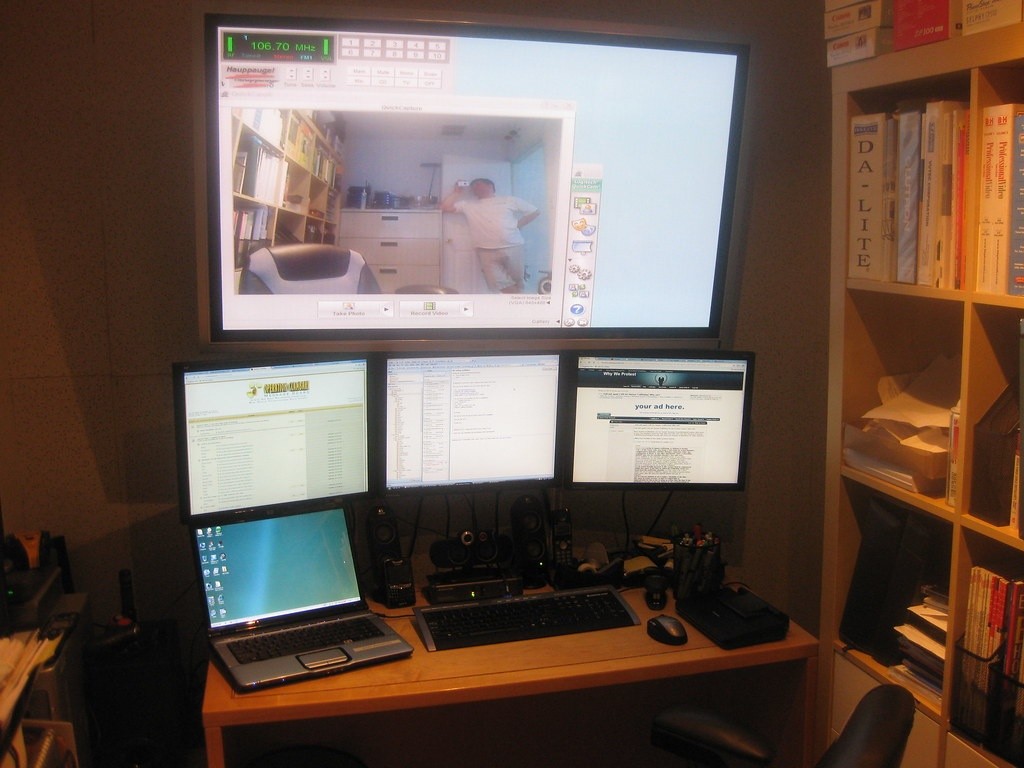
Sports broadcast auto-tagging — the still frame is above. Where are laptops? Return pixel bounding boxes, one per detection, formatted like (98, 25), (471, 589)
(191, 505), (414, 694)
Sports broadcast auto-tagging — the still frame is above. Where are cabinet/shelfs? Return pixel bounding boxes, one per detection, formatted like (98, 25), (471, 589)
(339, 208), (445, 296)
(231, 108), (346, 292)
(817, 20), (1024, 768)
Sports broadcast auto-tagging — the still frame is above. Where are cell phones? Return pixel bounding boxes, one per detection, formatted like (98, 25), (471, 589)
(384, 557), (416, 609)
(717, 589), (768, 618)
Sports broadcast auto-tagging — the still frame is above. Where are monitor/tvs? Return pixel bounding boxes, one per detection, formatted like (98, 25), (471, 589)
(173, 0), (758, 524)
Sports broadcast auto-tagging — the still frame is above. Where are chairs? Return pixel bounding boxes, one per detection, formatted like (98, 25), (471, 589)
(651, 683), (918, 768)
(238, 242), (386, 295)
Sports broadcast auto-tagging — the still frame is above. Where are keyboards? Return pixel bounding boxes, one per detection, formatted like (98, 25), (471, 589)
(412, 582), (641, 653)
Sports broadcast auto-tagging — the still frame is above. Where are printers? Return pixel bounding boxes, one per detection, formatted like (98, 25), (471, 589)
(6, 565), (88, 632)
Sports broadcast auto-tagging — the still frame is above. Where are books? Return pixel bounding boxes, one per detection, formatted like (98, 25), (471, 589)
(846, 100), (1024, 746)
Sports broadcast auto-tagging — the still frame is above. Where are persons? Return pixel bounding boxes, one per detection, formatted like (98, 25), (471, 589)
(441, 178), (541, 295)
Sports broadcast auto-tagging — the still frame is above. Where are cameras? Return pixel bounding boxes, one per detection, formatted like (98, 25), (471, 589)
(457, 181), (469, 187)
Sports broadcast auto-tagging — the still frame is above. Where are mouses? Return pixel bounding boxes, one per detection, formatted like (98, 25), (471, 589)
(647, 614), (688, 645)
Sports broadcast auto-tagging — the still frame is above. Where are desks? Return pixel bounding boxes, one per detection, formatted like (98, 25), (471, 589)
(203, 529), (822, 768)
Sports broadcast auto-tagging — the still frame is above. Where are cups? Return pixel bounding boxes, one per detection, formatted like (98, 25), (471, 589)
(458, 181), (469, 187)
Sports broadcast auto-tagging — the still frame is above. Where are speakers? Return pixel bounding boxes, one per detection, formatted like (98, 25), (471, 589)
(365, 505), (403, 602)
(511, 496), (551, 589)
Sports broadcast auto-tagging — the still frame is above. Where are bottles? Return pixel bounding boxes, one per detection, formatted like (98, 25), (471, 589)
(361, 189), (367, 209)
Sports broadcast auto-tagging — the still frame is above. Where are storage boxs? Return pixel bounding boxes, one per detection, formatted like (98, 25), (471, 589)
(824, 0), (863, 12)
(837, 496), (942, 667)
(973, 373), (1024, 526)
(826, 27), (893, 67)
(824, 0), (893, 39)
